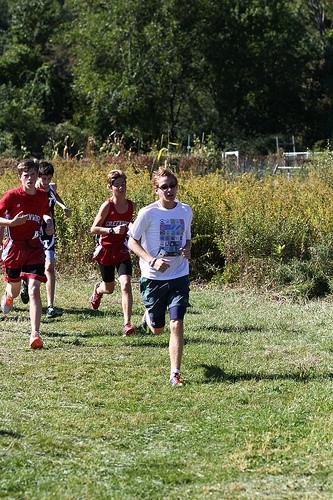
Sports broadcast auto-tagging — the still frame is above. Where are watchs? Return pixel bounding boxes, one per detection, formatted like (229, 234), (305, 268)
(109, 227), (114, 235)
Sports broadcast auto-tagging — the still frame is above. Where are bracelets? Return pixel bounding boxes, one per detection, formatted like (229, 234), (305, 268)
(11, 219), (14, 227)
(149, 258), (157, 267)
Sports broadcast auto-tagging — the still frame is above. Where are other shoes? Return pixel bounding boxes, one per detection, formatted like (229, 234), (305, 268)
(29, 331), (42, 349)
(1, 291), (12, 314)
(45, 306), (56, 318)
(141, 315), (149, 333)
(168, 372), (183, 387)
(89, 282), (102, 309)
(122, 321), (134, 337)
(19, 281), (29, 304)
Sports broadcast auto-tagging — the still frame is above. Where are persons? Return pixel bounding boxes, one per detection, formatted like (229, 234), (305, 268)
(19, 161), (73, 319)
(128, 168), (194, 387)
(91, 169), (136, 337)
(1, 157), (54, 350)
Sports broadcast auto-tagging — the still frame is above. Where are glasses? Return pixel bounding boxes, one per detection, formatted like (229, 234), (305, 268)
(157, 184), (176, 189)
